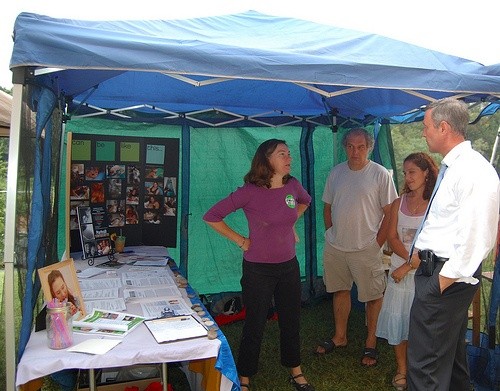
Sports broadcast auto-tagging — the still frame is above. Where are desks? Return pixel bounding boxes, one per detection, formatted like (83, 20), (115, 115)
(15, 246), (242, 391)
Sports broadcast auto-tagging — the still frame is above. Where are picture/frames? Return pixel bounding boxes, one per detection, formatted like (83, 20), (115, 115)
(37, 258), (87, 321)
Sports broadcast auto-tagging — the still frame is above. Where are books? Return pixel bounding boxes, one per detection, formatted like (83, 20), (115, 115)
(71, 307), (145, 338)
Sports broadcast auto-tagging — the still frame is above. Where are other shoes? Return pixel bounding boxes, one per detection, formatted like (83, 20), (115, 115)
(393, 372), (407, 391)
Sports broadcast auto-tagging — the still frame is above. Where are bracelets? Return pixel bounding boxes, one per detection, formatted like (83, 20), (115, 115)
(409, 264), (413, 269)
(240, 237), (245, 248)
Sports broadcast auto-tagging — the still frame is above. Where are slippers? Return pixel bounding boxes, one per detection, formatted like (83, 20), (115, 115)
(314, 337), (350, 356)
(360, 338), (379, 366)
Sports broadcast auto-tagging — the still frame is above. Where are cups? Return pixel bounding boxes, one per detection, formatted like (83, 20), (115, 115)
(114, 236), (127, 253)
(46, 302), (73, 350)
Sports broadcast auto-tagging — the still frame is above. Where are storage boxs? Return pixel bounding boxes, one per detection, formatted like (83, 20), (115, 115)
(77, 365), (162, 391)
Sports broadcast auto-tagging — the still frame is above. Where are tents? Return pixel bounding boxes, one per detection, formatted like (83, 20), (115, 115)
(5, 9), (500, 390)
(0, 90), (45, 138)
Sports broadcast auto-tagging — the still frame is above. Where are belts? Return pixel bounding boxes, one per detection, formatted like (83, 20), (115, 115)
(434, 257), (449, 261)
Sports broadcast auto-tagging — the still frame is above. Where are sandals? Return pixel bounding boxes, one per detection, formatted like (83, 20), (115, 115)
(289, 372), (315, 391)
(240, 384), (249, 391)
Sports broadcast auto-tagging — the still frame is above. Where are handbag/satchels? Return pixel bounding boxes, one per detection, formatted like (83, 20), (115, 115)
(201, 295), (216, 319)
(223, 296), (241, 316)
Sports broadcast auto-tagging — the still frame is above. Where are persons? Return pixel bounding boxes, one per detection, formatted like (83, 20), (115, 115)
(374, 152), (439, 389)
(407, 98), (500, 391)
(71, 163), (178, 258)
(203, 138), (315, 391)
(48, 270), (80, 316)
(315, 127), (400, 368)
